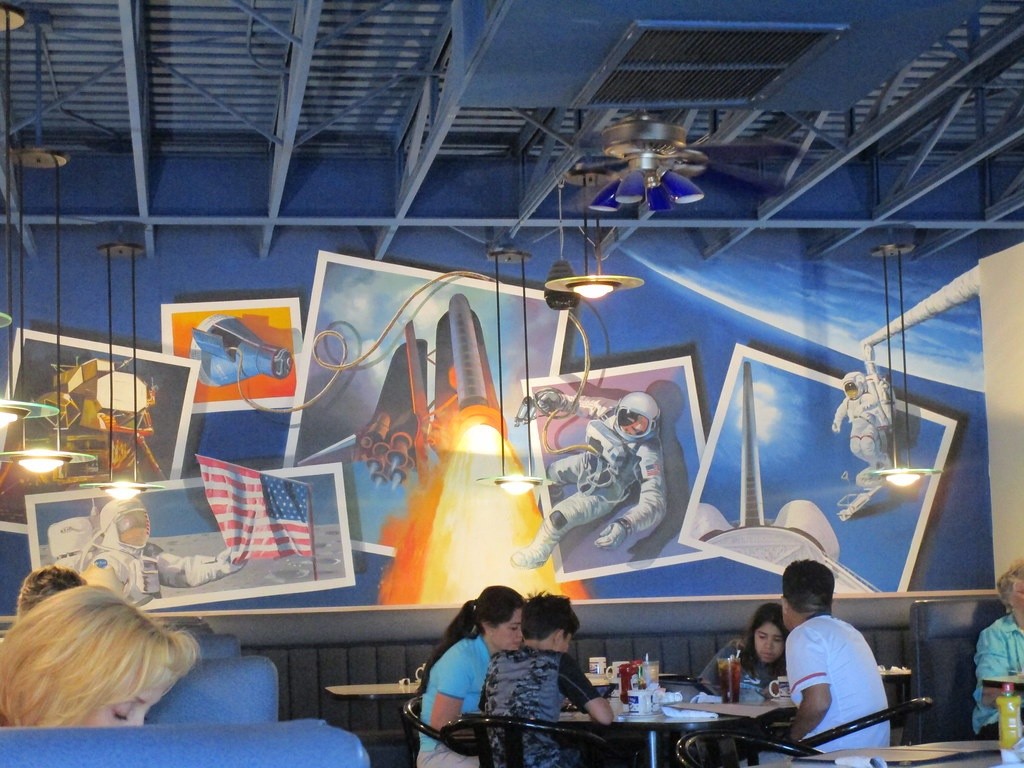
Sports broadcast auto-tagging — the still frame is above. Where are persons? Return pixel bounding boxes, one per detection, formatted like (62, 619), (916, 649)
(416, 586), (527, 768)
(832, 372), (895, 486)
(17, 564), (87, 624)
(669, 603), (791, 768)
(510, 387), (667, 570)
(88, 497), (249, 607)
(478, 591), (613, 768)
(972, 559), (1024, 740)
(721, 560), (890, 768)
(0, 584), (201, 728)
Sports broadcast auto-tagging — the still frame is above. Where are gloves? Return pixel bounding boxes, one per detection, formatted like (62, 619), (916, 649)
(593, 518), (632, 551)
(535, 391), (568, 414)
(212, 548), (248, 577)
(832, 419), (841, 433)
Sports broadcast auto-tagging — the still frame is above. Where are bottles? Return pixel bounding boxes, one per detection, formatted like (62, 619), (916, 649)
(996, 683), (1023, 752)
(619, 663), (639, 710)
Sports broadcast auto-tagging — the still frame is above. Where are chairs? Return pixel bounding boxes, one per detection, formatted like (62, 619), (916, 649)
(402, 697), (941, 768)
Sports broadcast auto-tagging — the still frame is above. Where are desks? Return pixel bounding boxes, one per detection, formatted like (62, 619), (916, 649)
(981, 675), (1024, 691)
(552, 700), (797, 768)
(874, 666), (912, 749)
(326, 672), (672, 701)
(743, 740), (1024, 768)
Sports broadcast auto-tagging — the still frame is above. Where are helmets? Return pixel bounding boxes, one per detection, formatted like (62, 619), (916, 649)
(614, 392), (661, 442)
(842, 372), (867, 400)
(99, 498), (151, 556)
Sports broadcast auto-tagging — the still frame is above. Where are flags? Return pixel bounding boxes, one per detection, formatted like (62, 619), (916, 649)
(196, 454), (313, 564)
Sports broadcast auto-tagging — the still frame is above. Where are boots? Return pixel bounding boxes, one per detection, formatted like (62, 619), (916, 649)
(511, 526), (563, 570)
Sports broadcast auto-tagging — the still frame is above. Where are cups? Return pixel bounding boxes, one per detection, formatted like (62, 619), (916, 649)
(642, 661), (660, 688)
(416, 664), (426, 681)
(627, 689), (666, 714)
(607, 661), (629, 683)
(718, 658), (741, 703)
(589, 657), (606, 675)
(769, 676), (791, 699)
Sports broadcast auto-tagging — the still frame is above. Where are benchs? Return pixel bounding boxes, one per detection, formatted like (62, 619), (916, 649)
(1, 617), (373, 768)
(902, 598), (999, 746)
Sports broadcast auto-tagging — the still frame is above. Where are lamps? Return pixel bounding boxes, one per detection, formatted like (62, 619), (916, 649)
(873, 239), (941, 486)
(0, 4), (59, 429)
(0, 140), (97, 476)
(81, 238), (166, 502)
(588, 168), (705, 212)
(544, 133), (646, 298)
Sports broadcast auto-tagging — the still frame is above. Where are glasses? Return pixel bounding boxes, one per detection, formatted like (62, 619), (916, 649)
(117, 511), (147, 534)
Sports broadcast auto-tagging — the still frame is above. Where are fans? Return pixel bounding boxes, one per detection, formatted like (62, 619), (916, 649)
(573, 108), (802, 197)
